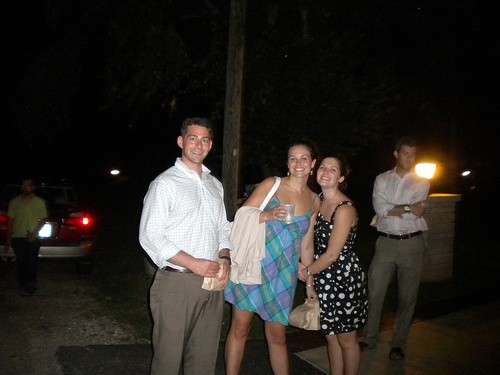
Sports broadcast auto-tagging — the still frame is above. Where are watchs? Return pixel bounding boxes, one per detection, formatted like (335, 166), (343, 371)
(404, 204), (410, 214)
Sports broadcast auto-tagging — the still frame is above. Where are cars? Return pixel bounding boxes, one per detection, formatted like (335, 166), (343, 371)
(0, 170), (98, 275)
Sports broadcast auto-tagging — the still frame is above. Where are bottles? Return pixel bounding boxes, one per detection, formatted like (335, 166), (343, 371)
(27, 230), (34, 242)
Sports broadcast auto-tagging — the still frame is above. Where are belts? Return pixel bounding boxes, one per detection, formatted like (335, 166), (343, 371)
(162, 266), (192, 272)
(378, 231), (423, 239)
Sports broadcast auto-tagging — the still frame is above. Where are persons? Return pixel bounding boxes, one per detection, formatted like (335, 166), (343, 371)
(139, 119), (234, 375)
(297, 154), (370, 375)
(6, 179), (48, 299)
(224, 140), (321, 375)
(358, 135), (431, 360)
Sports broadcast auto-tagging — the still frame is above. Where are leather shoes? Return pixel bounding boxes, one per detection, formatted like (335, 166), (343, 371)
(359, 342), (376, 351)
(389, 348), (404, 359)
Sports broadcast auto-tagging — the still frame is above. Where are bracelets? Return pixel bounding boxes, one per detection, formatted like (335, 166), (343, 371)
(306, 266), (313, 277)
(220, 256), (232, 266)
(306, 282), (315, 288)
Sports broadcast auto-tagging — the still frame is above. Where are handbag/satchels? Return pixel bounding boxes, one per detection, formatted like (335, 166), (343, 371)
(289, 299), (321, 330)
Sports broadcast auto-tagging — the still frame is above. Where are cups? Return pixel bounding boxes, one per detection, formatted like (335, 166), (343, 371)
(281, 204), (295, 225)
